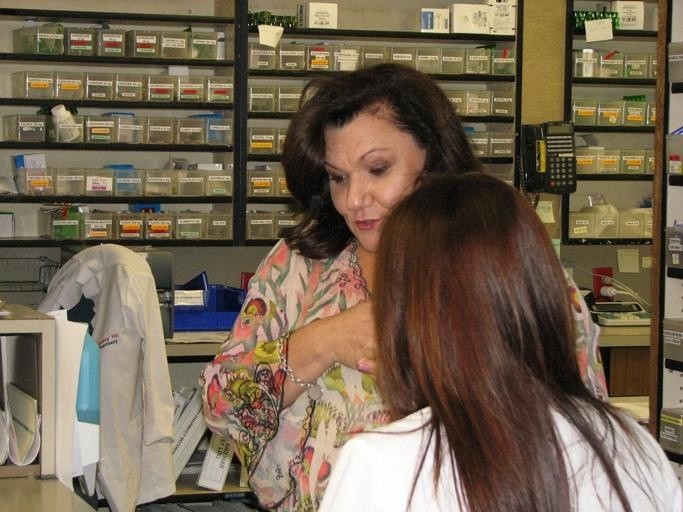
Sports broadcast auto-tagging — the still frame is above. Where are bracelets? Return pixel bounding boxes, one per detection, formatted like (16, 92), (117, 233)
(275, 331), (326, 400)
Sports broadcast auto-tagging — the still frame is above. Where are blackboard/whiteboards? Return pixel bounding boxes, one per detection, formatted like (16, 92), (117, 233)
(522, 121), (577, 194)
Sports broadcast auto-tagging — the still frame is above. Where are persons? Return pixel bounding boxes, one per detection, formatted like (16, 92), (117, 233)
(316, 168), (681, 511)
(202, 62), (612, 512)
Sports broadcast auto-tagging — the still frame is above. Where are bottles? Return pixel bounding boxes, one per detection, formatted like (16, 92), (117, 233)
(50, 104), (81, 142)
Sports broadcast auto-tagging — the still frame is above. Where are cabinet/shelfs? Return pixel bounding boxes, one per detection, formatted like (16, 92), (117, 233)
(649, 1), (683, 466)
